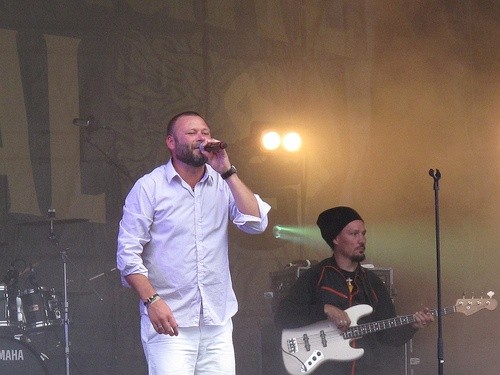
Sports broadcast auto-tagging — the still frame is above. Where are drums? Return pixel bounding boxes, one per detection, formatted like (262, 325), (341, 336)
(13, 287), (69, 331)
(0, 336), (51, 375)
(0, 285), (13, 327)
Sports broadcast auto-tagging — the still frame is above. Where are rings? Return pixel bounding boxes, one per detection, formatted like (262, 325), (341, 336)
(344, 320), (348, 323)
(158, 325), (164, 329)
(339, 320), (343, 323)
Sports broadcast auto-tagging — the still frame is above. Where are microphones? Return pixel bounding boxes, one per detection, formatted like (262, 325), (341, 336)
(73, 119), (107, 130)
(204, 142), (227, 152)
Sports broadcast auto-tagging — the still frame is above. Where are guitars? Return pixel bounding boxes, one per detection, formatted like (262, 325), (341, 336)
(279, 288), (500, 375)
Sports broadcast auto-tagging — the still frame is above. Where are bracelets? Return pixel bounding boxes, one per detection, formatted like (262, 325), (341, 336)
(143, 294), (160, 307)
(220, 164), (237, 179)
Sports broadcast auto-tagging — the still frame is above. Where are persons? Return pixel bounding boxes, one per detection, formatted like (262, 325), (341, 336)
(275, 206), (434, 375)
(117, 111), (272, 375)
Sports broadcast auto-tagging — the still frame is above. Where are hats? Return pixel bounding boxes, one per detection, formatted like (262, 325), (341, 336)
(317, 207), (364, 245)
